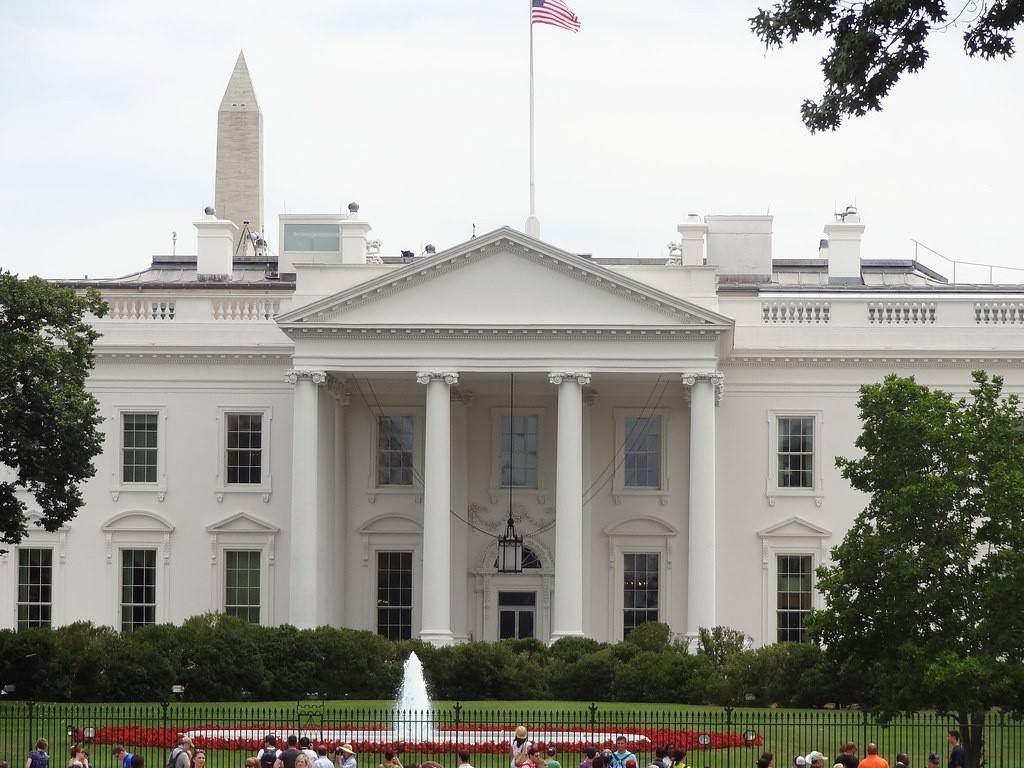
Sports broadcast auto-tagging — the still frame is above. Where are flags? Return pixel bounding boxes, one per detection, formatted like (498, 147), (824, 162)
(531, 0), (581, 34)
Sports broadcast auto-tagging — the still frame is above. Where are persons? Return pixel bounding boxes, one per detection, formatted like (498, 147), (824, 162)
(169, 737), (205, 768)
(25, 738), (49, 768)
(579, 736), (638, 768)
(758, 752), (774, 768)
(793, 751), (828, 768)
(69, 746), (92, 768)
(509, 725), (561, 768)
(647, 743), (692, 768)
(112, 747), (145, 768)
(925, 753), (941, 768)
(833, 742), (911, 768)
(948, 730), (965, 768)
(377, 750), (443, 768)
(245, 735), (357, 768)
(458, 750), (474, 768)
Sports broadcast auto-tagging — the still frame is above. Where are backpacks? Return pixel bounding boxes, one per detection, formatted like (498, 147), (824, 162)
(165, 747), (187, 768)
(261, 748), (279, 768)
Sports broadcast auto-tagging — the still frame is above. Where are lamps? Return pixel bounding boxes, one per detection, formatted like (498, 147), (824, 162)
(172, 680), (185, 693)
(744, 692), (756, 700)
(699, 734), (711, 751)
(497, 369), (525, 570)
(744, 730), (756, 748)
(4, 684), (17, 692)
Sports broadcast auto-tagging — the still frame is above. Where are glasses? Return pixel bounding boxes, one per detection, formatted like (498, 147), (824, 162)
(668, 748), (674, 750)
(116, 753), (119, 758)
(533, 755), (540, 757)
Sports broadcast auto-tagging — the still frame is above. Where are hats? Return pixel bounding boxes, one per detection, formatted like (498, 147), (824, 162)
(516, 726), (527, 739)
(812, 752), (828, 760)
(181, 737), (194, 747)
(805, 751), (818, 764)
(600, 749), (612, 762)
(833, 763), (843, 768)
(548, 747), (556, 754)
(340, 743), (356, 755)
(626, 760), (636, 768)
(796, 756), (805, 765)
(929, 754), (940, 763)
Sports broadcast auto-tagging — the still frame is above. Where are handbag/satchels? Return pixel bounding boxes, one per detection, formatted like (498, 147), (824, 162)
(515, 741), (528, 767)
